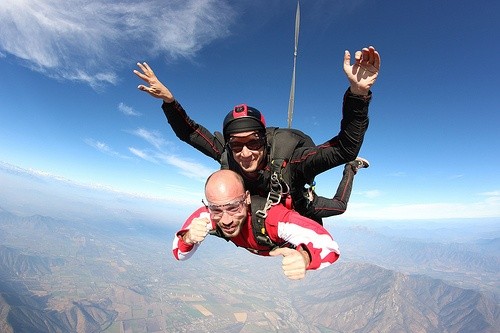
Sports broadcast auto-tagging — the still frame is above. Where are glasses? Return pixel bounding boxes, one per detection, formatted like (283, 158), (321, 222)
(207, 196), (247, 214)
(225, 133), (264, 153)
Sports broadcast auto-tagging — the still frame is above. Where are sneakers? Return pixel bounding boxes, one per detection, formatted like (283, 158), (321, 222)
(346, 156), (370, 174)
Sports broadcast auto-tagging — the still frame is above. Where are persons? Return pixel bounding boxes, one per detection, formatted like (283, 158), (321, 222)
(133, 46), (381, 228)
(173, 171), (340, 280)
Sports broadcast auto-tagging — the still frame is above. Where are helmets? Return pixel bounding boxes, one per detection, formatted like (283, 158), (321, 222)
(223, 104), (267, 136)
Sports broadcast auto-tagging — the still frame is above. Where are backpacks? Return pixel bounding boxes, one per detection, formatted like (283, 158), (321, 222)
(220, 125), (317, 208)
